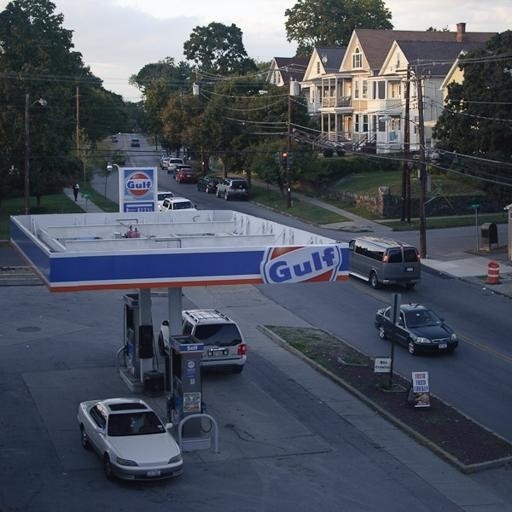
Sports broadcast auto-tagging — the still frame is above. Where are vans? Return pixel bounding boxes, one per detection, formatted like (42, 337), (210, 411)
(346, 235), (421, 290)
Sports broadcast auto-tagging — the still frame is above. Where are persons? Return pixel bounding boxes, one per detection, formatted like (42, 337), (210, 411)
(72, 184), (79, 203)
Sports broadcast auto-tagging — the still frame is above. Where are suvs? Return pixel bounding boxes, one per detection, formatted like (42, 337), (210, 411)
(183, 306), (247, 373)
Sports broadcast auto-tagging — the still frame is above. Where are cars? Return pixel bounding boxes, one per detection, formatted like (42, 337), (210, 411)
(375, 303), (457, 357)
(77, 396), (182, 484)
(153, 153), (249, 213)
(112, 135), (119, 143)
(131, 138), (141, 148)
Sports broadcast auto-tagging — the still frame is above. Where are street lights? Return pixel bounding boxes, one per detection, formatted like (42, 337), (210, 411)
(178, 93), (206, 177)
(377, 111), (427, 258)
(24, 94), (49, 211)
(105, 162), (120, 172)
(257, 89), (301, 209)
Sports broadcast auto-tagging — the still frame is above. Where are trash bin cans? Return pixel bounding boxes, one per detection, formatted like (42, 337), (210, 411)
(480, 223), (498, 253)
